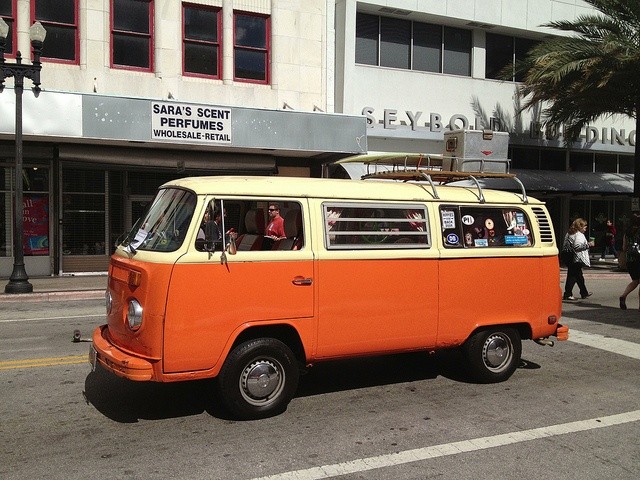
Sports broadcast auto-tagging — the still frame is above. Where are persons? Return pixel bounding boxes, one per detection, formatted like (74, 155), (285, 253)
(562, 218), (595, 300)
(265, 205), (287, 241)
(620, 213), (640, 309)
(599, 219), (618, 262)
(205, 206), (239, 245)
(200, 208), (210, 233)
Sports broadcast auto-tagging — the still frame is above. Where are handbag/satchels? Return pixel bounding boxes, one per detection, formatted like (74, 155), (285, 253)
(560, 231), (577, 267)
(618, 232), (629, 271)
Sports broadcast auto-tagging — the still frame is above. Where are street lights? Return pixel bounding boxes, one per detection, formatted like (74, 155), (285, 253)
(0, 16), (47, 293)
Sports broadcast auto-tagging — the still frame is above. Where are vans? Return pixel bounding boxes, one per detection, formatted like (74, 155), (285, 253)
(88, 128), (570, 417)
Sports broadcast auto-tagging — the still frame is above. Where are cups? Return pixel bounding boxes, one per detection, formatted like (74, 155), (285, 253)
(589, 237), (595, 243)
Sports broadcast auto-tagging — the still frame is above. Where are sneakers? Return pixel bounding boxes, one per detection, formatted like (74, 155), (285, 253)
(564, 295), (575, 300)
(582, 292), (592, 299)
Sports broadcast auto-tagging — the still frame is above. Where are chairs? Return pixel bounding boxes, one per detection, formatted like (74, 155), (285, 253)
(235, 209), (265, 251)
(269, 210), (302, 250)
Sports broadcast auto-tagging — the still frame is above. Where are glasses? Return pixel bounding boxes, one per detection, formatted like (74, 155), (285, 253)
(269, 209), (277, 211)
